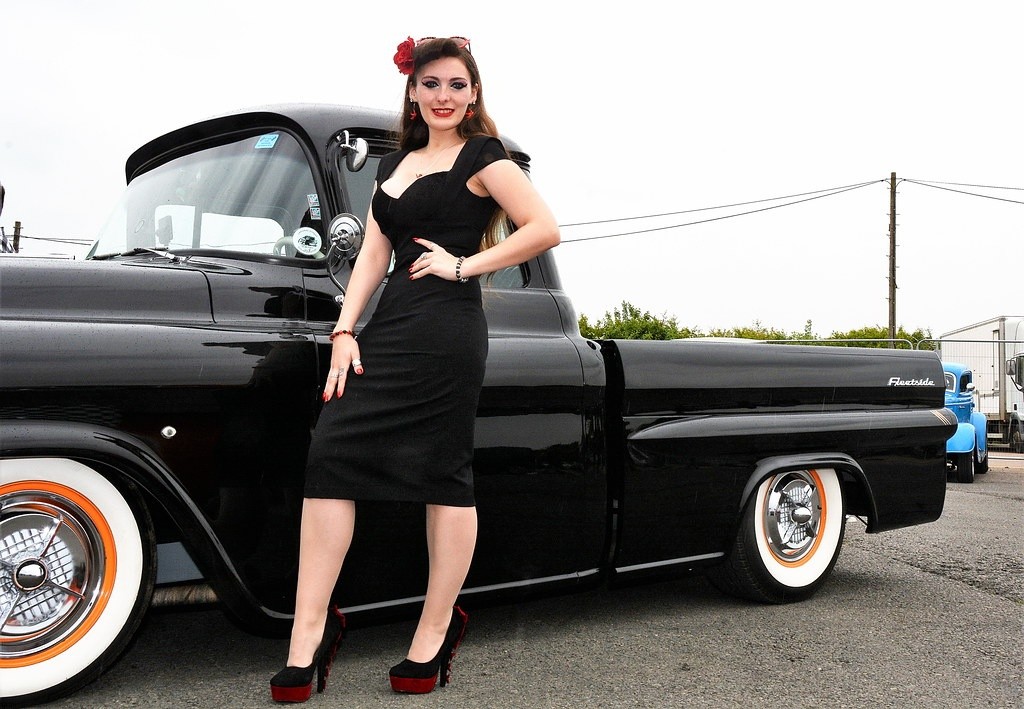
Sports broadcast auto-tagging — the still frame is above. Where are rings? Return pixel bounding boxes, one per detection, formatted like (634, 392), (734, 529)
(421, 253), (427, 259)
(339, 368), (345, 376)
(429, 244), (437, 250)
(332, 374), (338, 378)
(352, 359), (361, 366)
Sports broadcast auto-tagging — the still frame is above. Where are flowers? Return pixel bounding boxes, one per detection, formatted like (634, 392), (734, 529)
(393, 35), (416, 77)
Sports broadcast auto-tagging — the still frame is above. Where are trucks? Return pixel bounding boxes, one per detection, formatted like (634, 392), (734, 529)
(938, 315), (1024, 453)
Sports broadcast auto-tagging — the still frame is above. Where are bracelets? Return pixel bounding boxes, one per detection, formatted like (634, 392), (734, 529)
(456, 256), (469, 283)
(329, 329), (356, 341)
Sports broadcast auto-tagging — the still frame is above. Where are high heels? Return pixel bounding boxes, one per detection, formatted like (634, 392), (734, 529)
(270, 606), (346, 704)
(389, 603), (467, 694)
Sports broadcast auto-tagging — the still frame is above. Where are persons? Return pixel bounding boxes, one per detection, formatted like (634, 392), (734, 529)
(270, 36), (561, 702)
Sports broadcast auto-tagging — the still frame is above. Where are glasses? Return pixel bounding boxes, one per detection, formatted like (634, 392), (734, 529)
(415, 36), (472, 53)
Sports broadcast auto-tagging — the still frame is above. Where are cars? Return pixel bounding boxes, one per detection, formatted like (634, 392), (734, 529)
(940, 361), (989, 483)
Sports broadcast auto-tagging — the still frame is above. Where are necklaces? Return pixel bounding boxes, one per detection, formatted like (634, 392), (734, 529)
(415, 141), (458, 178)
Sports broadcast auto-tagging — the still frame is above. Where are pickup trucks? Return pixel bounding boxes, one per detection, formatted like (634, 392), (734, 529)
(1, 102), (948, 708)
